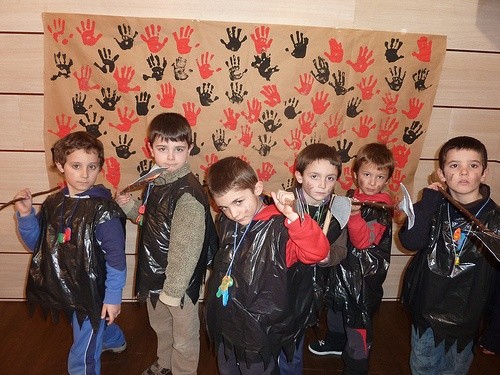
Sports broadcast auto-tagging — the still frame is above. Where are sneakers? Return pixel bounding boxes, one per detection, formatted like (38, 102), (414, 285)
(308, 337), (343, 356)
(102, 339), (128, 353)
(140, 361), (171, 375)
